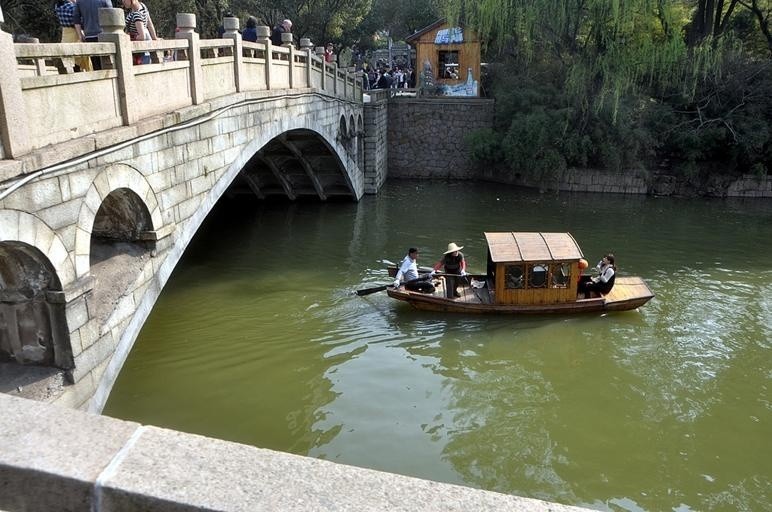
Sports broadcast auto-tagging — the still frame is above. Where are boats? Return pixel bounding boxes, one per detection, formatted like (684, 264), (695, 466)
(385, 232), (655, 314)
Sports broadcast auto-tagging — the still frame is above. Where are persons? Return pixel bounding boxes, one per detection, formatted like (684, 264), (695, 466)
(357, 58), (417, 91)
(429, 241), (466, 298)
(270, 19), (293, 47)
(120, 0), (165, 66)
(71, 0), (115, 72)
(53, 0), (95, 75)
(323, 42), (335, 62)
(242, 16), (259, 43)
(395, 247), (436, 294)
(217, 13), (243, 42)
(583, 252), (616, 299)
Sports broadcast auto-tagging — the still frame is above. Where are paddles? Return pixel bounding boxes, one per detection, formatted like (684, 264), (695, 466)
(357, 275), (437, 296)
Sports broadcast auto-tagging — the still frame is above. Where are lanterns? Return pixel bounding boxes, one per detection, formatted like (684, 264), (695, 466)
(577, 259), (589, 276)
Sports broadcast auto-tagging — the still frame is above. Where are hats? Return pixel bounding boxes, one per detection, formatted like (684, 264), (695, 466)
(443, 243), (464, 255)
